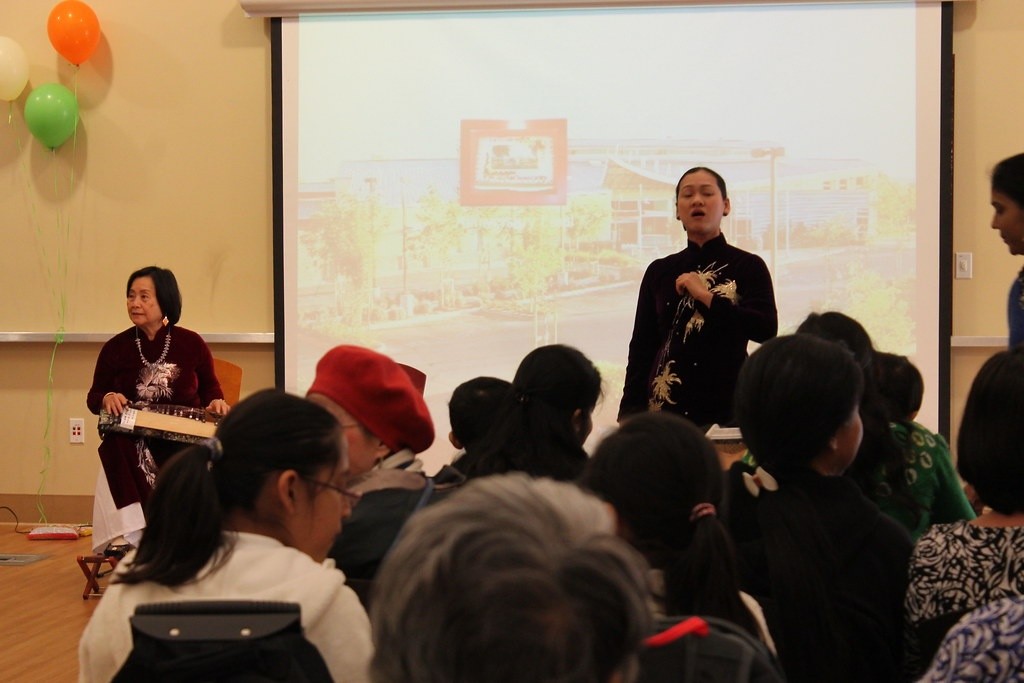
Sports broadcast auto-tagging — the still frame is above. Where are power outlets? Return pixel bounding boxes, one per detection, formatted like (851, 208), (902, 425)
(956, 253), (973, 279)
(69, 418), (84, 443)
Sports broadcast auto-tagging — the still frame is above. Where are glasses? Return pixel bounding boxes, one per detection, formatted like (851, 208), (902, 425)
(301, 476), (363, 507)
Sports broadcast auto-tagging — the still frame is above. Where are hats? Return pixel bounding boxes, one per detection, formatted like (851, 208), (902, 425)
(306, 344), (434, 452)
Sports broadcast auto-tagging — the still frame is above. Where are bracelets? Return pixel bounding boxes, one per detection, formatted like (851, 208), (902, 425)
(103, 392), (116, 405)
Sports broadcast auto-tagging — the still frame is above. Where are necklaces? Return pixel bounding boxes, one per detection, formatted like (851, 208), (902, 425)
(135, 326), (171, 370)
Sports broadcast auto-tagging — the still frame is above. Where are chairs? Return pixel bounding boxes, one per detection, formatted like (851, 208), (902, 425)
(76, 358), (243, 598)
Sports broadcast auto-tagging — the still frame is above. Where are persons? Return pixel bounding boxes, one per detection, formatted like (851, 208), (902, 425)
(578, 411), (777, 652)
(717, 333), (913, 683)
(304, 345), (449, 615)
(902, 340), (1024, 683)
(990, 152), (1024, 349)
(615, 167), (778, 428)
(79, 388), (376, 683)
(796, 311), (977, 541)
(449, 344), (602, 481)
(369, 471), (658, 683)
(87, 266), (231, 556)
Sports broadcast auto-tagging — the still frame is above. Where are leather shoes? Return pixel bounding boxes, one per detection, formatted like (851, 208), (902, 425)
(105, 543), (129, 557)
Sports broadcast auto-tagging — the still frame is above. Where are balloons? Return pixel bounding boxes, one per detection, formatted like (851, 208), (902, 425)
(23, 82), (79, 148)
(47, 0), (101, 67)
(0, 36), (29, 101)
